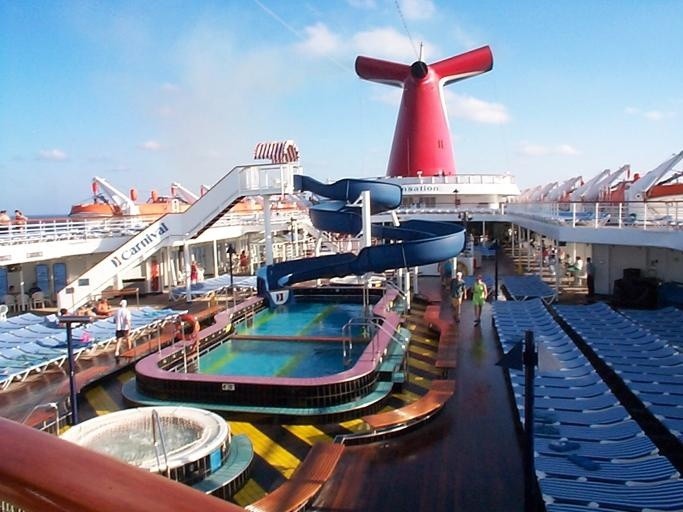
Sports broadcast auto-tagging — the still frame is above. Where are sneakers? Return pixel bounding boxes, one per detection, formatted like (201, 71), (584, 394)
(453, 318), (482, 328)
(114, 355), (120, 359)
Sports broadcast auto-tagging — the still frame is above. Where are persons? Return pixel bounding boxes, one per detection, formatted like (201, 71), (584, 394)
(470, 272), (487, 328)
(6, 283), (23, 303)
(238, 250), (249, 274)
(26, 281), (42, 298)
(448, 269), (467, 322)
(0, 210), (10, 234)
(113, 298), (132, 358)
(442, 259), (454, 291)
(503, 224), (594, 299)
(59, 296), (112, 326)
(437, 258), (447, 288)
(190, 259), (198, 287)
(13, 209), (27, 233)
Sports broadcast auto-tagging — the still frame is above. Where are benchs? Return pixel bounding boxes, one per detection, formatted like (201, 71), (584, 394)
(359, 379), (457, 440)
(243, 437), (347, 512)
(417, 286), (459, 379)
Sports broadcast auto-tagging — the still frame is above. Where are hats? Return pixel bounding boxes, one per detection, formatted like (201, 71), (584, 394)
(456, 272), (462, 276)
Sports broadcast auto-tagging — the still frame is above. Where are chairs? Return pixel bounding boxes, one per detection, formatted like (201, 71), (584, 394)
(1, 292), (189, 393)
(168, 273), (257, 308)
(463, 245), (682, 512)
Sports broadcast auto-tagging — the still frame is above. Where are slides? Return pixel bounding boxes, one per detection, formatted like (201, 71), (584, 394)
(258, 175), (466, 308)
